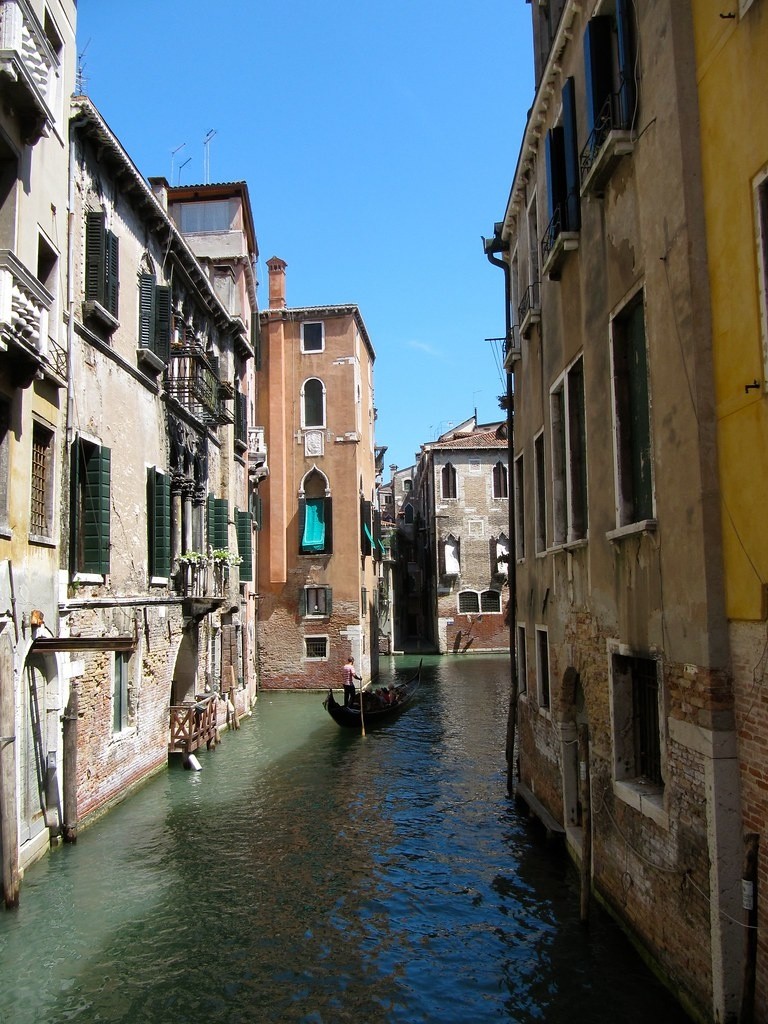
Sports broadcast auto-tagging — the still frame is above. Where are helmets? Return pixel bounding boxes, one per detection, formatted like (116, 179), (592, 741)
(348, 657), (354, 662)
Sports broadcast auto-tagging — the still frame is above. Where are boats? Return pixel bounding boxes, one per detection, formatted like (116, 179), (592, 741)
(323, 657), (423, 729)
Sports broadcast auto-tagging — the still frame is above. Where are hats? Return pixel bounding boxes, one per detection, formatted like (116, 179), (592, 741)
(382, 688), (387, 693)
(376, 688), (381, 696)
(365, 686), (372, 693)
(388, 682), (394, 689)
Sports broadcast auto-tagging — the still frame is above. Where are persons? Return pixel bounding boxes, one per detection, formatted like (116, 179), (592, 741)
(359, 683), (405, 711)
(340, 656), (362, 708)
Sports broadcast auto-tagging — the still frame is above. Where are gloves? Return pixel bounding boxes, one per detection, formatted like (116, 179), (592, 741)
(355, 676), (362, 680)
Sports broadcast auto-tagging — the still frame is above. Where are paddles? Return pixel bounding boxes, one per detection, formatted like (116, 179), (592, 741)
(360, 675), (367, 737)
